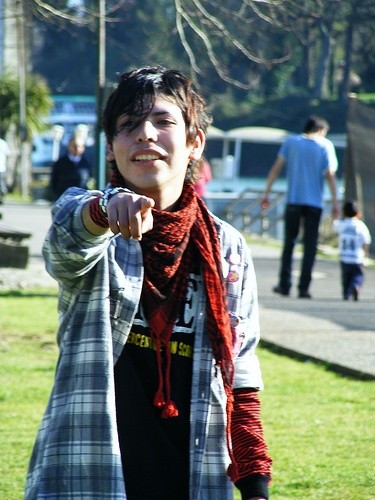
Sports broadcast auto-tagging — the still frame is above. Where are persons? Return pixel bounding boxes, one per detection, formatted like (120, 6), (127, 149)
(259, 113), (340, 300)
(21, 65), (273, 500)
(0, 138), (11, 205)
(46, 135), (91, 204)
(330, 198), (372, 302)
(4, 121), (20, 194)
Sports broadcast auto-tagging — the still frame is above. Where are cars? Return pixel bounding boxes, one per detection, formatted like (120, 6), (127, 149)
(24, 93), (350, 211)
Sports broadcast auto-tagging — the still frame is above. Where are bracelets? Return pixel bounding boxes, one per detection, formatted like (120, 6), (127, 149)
(99, 187), (134, 215)
(88, 195), (110, 228)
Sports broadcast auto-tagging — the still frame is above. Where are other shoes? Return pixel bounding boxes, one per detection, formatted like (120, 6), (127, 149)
(299, 293), (311, 298)
(273, 287), (289, 296)
(352, 288), (358, 300)
